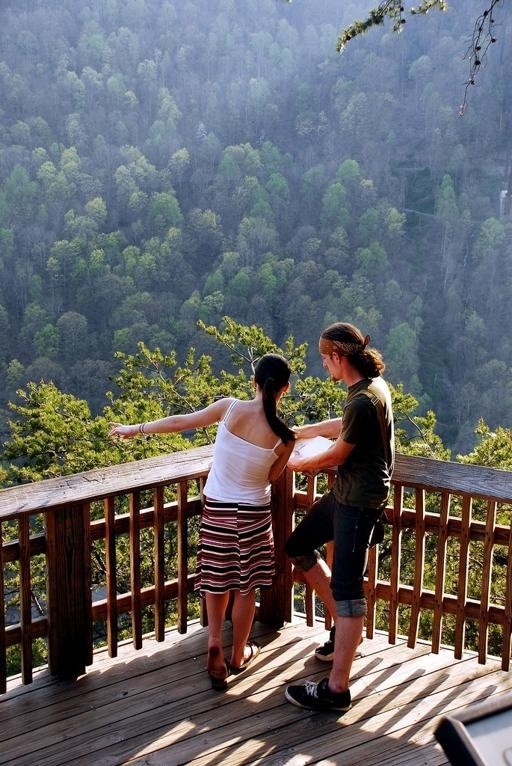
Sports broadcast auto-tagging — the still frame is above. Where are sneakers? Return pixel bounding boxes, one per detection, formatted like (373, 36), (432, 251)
(285, 678), (351, 711)
(316, 626), (363, 663)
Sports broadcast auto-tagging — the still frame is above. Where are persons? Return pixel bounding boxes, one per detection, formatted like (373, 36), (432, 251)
(104, 352), (299, 687)
(281, 319), (397, 715)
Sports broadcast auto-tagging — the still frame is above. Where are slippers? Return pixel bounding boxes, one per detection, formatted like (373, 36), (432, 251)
(208, 658), (228, 693)
(229, 641), (261, 669)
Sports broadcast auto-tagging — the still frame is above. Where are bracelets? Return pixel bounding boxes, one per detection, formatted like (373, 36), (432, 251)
(137, 423), (147, 434)
